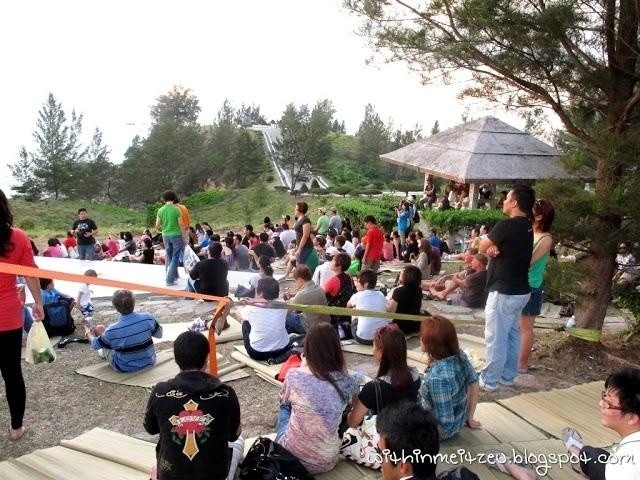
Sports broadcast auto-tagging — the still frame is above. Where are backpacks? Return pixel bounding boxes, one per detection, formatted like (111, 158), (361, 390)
(41, 295), (77, 336)
(435, 464), (481, 480)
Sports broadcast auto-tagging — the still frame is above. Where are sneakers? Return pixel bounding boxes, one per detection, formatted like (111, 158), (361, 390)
(97, 348), (104, 358)
(8, 425), (26, 441)
(496, 377), (513, 386)
(477, 374), (499, 392)
(514, 367), (529, 374)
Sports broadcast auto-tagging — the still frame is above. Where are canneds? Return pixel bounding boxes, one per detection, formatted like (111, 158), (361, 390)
(38, 347), (54, 363)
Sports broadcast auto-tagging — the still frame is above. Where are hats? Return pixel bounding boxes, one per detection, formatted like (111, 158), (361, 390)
(323, 247), (341, 258)
(318, 205), (337, 215)
(472, 253), (489, 266)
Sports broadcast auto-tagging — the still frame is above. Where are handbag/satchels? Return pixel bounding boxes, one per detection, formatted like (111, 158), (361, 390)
(337, 379), (383, 473)
(240, 435), (317, 480)
(347, 259), (361, 276)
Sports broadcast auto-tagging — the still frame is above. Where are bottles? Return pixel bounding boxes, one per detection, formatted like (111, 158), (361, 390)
(337, 326), (346, 338)
(566, 315), (575, 328)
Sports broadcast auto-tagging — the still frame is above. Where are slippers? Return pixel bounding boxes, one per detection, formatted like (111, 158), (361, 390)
(560, 426), (585, 474)
(482, 448), (533, 476)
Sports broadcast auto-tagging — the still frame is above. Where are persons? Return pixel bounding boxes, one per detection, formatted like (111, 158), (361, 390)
(142, 330), (245, 480)
(339, 326), (421, 470)
(472, 184), (536, 391)
(274, 321), (361, 475)
(0, 190), (45, 440)
(517, 199), (555, 375)
(417, 314), (483, 442)
(612, 241), (639, 301)
(375, 400), (454, 480)
(483, 367), (640, 480)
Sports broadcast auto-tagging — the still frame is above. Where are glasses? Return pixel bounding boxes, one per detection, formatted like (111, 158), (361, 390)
(600, 390), (624, 412)
(373, 442), (392, 460)
(535, 197), (544, 213)
